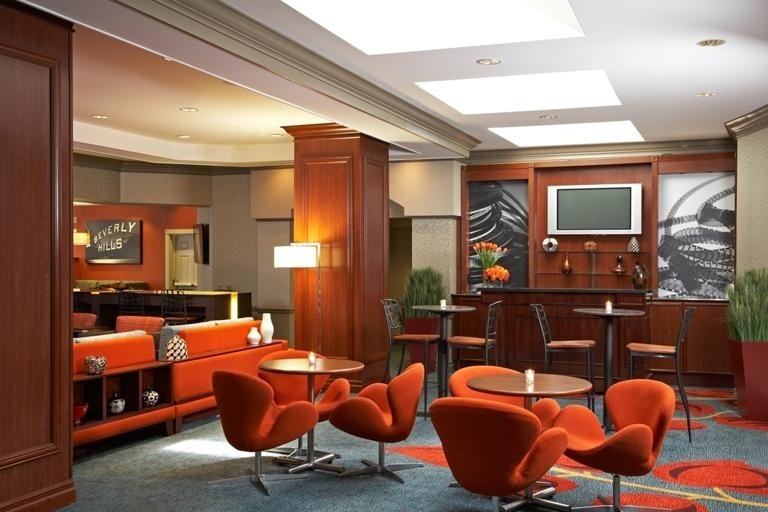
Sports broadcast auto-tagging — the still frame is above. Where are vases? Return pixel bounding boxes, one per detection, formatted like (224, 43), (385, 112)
(246, 327), (261, 344)
(260, 313), (274, 344)
(482, 269), (488, 287)
(488, 280), (503, 288)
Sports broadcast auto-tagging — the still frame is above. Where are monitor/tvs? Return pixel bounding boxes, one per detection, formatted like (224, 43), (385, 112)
(546, 182), (644, 237)
(192, 223), (207, 266)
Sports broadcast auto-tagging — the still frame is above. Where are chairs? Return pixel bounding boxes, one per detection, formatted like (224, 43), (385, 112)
(328, 360), (429, 487)
(160, 288), (199, 325)
(530, 304), (596, 413)
(117, 290), (146, 315)
(251, 350), (345, 464)
(445, 362), (553, 489)
(211, 366), (313, 497)
(447, 300), (503, 390)
(379, 298), (440, 420)
(427, 395), (571, 506)
(553, 375), (676, 510)
(626, 307), (695, 443)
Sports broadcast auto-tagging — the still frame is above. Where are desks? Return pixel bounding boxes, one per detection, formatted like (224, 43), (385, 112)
(73, 288), (238, 319)
(411, 304), (476, 398)
(464, 372), (594, 510)
(257, 356), (368, 475)
(573, 308), (645, 436)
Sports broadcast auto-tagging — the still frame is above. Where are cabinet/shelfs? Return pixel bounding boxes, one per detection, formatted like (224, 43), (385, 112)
(73, 340), (288, 448)
(536, 249), (651, 289)
(647, 301), (737, 388)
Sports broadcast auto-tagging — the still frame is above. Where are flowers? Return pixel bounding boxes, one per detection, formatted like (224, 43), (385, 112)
(473, 241), (497, 268)
(485, 264), (511, 282)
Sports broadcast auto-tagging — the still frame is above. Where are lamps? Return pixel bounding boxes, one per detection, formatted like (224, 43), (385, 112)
(273, 245), (317, 268)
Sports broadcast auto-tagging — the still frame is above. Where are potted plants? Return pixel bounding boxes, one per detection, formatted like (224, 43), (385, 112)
(725, 267), (768, 421)
(399, 266), (444, 374)
